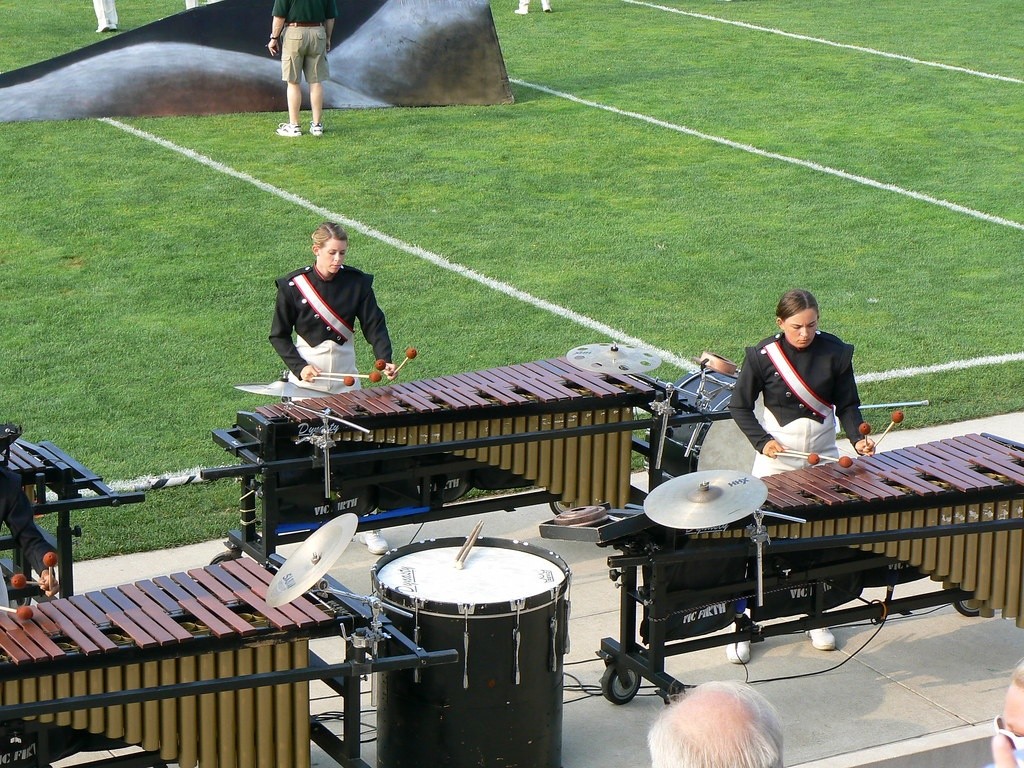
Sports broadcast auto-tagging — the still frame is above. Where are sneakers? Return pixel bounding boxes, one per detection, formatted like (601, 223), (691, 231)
(805, 626), (836, 650)
(514, 10), (528, 15)
(359, 529), (389, 555)
(726, 622), (752, 663)
(309, 121), (323, 136)
(543, 7), (553, 13)
(275, 122), (303, 137)
(95, 23), (117, 32)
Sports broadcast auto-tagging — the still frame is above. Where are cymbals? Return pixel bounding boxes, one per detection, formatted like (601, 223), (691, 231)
(566, 341), (663, 374)
(643, 469), (769, 530)
(233, 379), (334, 399)
(265, 512), (359, 607)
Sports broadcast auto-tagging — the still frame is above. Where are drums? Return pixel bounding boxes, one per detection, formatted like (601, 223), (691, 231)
(370, 533), (573, 768)
(643, 368), (764, 484)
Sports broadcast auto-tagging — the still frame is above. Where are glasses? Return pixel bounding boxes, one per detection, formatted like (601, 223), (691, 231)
(994, 714), (1024, 752)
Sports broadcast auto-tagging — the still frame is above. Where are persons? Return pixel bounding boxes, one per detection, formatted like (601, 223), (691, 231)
(725, 290), (876, 664)
(990, 662), (1024, 768)
(515, 0), (552, 14)
(93, 0), (118, 32)
(267, 0), (338, 137)
(647, 680), (784, 768)
(0, 464), (60, 613)
(269, 222), (398, 555)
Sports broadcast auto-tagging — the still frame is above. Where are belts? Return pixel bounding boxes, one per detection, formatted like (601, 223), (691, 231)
(285, 21), (326, 27)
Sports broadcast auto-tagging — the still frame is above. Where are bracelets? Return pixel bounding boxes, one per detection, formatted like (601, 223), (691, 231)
(270, 33), (278, 39)
